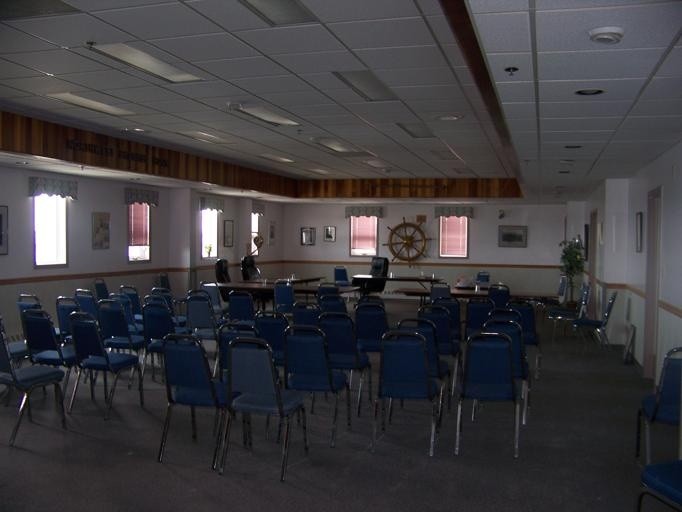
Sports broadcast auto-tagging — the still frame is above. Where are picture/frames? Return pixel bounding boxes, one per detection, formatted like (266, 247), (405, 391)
(324, 226), (336, 242)
(0, 205), (8, 255)
(224, 220), (233, 247)
(635, 213), (643, 252)
(92, 212), (111, 249)
(635, 212), (642, 252)
(498, 225), (527, 248)
